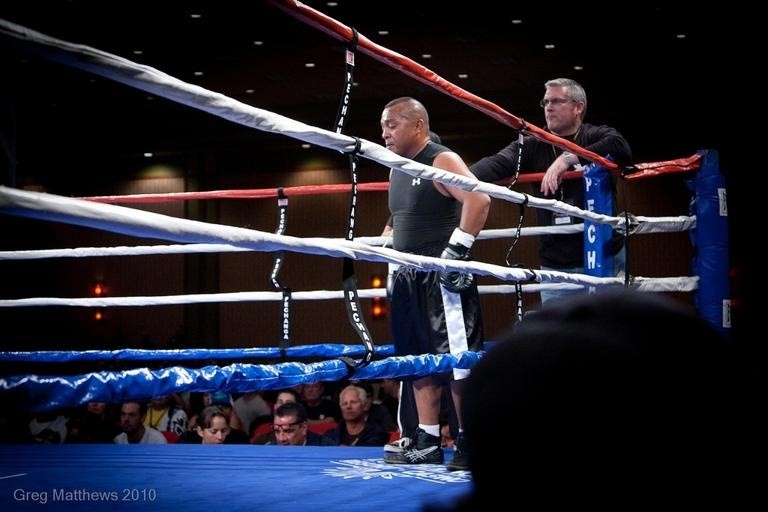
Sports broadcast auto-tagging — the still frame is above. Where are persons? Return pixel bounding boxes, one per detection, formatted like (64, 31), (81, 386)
(468, 78), (632, 307)
(459, 290), (768, 512)
(0, 377), (455, 448)
(380, 97), (491, 471)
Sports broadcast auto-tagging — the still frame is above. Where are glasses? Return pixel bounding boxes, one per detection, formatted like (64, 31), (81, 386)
(540, 99), (577, 108)
(271, 420), (304, 433)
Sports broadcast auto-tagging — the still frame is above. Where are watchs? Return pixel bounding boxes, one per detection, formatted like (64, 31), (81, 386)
(563, 150), (572, 165)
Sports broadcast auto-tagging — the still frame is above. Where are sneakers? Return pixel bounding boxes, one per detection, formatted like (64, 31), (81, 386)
(447, 434), (473, 472)
(383, 428), (444, 464)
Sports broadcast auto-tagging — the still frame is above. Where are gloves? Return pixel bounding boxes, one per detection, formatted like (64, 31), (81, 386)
(434, 247), (474, 293)
(382, 244), (394, 249)
